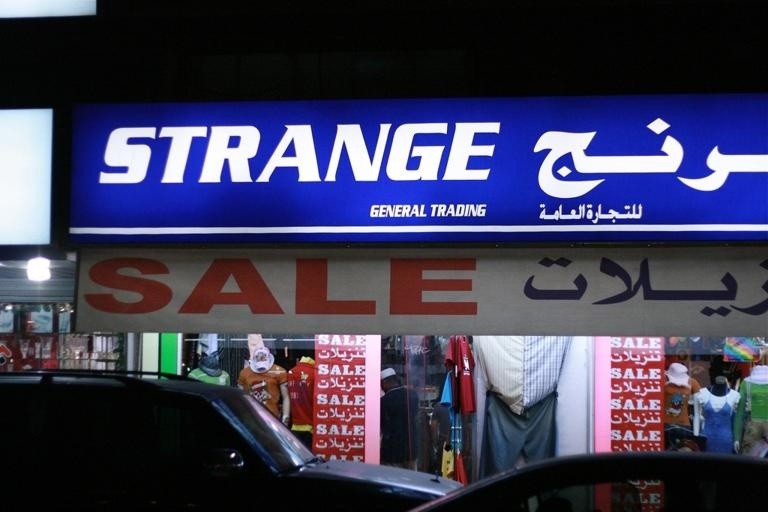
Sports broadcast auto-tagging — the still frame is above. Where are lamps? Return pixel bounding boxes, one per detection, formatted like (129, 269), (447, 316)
(28, 257), (51, 281)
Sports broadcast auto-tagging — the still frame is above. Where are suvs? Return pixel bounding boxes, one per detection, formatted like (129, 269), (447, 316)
(1, 367), (466, 509)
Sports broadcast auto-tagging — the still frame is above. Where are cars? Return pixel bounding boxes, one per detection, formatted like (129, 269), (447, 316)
(409, 449), (766, 510)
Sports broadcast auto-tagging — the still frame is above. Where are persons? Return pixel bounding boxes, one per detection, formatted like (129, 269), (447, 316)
(732, 364), (768, 456)
(238, 346), (290, 426)
(187, 356), (231, 387)
(380, 368), (419, 472)
(287, 356), (315, 451)
(694, 375), (741, 454)
(665, 362), (700, 426)
(430, 403), (470, 478)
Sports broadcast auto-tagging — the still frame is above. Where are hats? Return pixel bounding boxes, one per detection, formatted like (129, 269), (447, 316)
(664, 363), (690, 388)
(198, 353), (223, 377)
(250, 347), (275, 374)
(380, 367), (397, 380)
